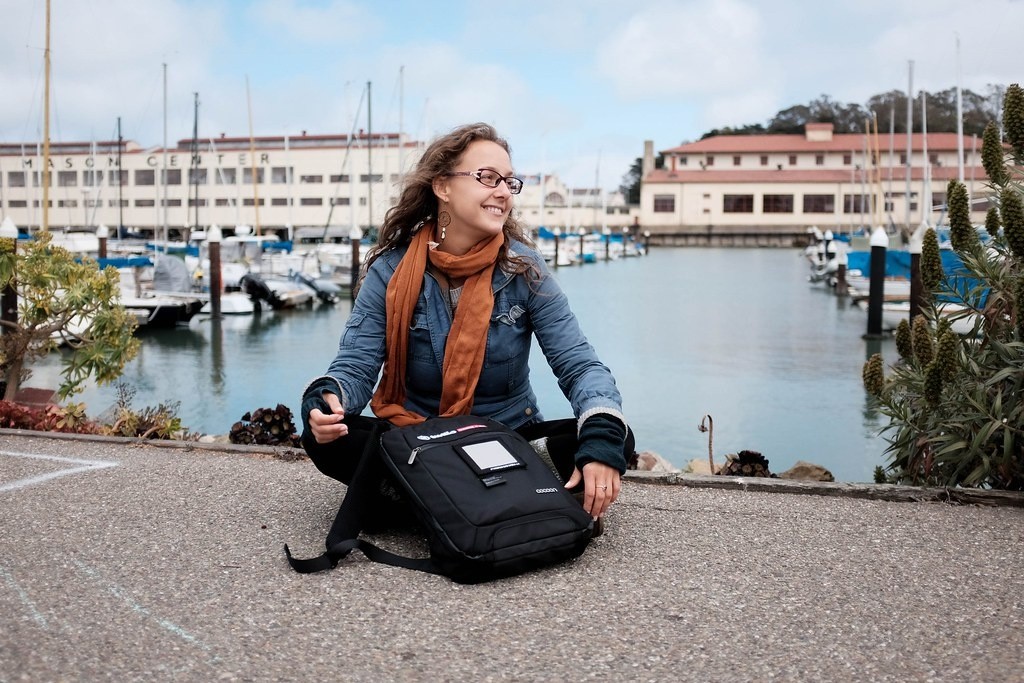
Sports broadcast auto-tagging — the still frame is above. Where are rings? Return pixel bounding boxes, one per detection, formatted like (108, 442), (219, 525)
(597, 486), (608, 491)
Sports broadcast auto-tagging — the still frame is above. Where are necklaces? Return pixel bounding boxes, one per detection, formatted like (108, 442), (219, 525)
(443, 274), (456, 290)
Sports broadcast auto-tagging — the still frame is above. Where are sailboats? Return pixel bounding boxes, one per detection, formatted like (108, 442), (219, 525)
(520, 158), (647, 267)
(0, 1), (409, 357)
(800, 36), (1016, 337)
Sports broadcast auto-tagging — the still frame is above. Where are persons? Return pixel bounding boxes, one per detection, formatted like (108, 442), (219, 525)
(297, 123), (636, 521)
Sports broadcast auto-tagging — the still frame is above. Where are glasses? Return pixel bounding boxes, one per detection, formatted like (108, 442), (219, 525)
(442, 168), (523, 195)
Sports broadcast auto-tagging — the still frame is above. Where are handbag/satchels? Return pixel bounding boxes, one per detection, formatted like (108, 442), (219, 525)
(378, 413), (593, 584)
